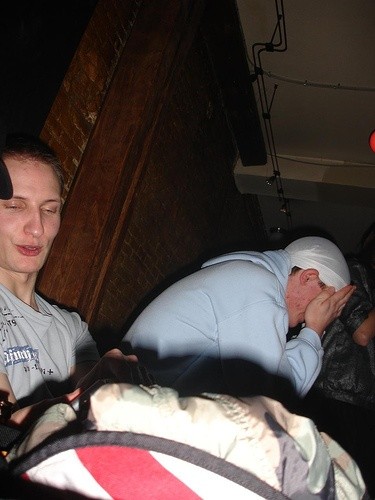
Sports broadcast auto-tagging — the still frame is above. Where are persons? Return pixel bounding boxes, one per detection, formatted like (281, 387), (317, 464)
(120, 224), (375, 500)
(0, 136), (157, 463)
